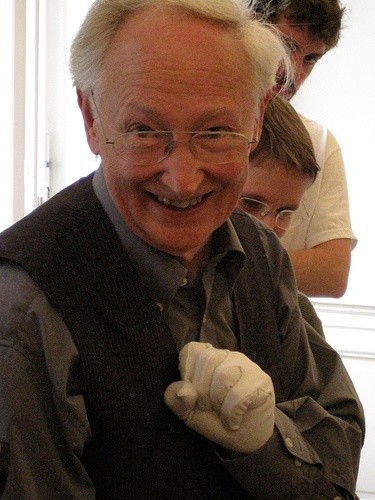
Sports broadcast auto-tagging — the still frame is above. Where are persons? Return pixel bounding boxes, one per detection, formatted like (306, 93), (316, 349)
(245, 0), (358, 299)
(0, 0), (366, 500)
(235, 90), (321, 241)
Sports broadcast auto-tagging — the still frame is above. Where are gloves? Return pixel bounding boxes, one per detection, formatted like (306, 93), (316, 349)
(163, 340), (277, 455)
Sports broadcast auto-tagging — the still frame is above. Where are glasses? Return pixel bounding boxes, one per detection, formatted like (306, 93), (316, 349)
(237, 196), (310, 232)
(89, 89), (261, 167)
